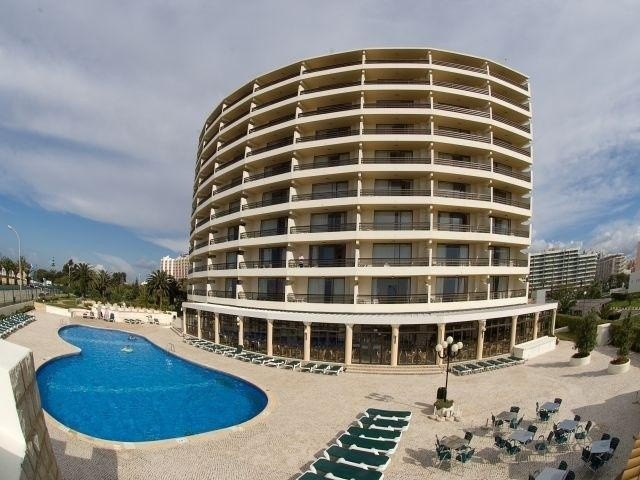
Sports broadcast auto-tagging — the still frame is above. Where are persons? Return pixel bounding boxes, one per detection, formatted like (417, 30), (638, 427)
(298, 253), (305, 268)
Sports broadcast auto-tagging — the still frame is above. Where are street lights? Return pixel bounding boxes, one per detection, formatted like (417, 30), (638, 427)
(436, 336), (464, 403)
(7, 225), (22, 302)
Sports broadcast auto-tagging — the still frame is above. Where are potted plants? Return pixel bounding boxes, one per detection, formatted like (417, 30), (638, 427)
(607, 309), (637, 375)
(569, 306), (598, 366)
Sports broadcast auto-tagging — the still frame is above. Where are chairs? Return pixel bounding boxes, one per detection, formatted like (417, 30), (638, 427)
(451, 356), (525, 377)
(0, 312), (36, 340)
(124, 315), (161, 328)
(435, 397), (620, 480)
(185, 337), (344, 377)
(82, 311), (115, 323)
(296, 406), (412, 480)
(363, 298), (378, 305)
